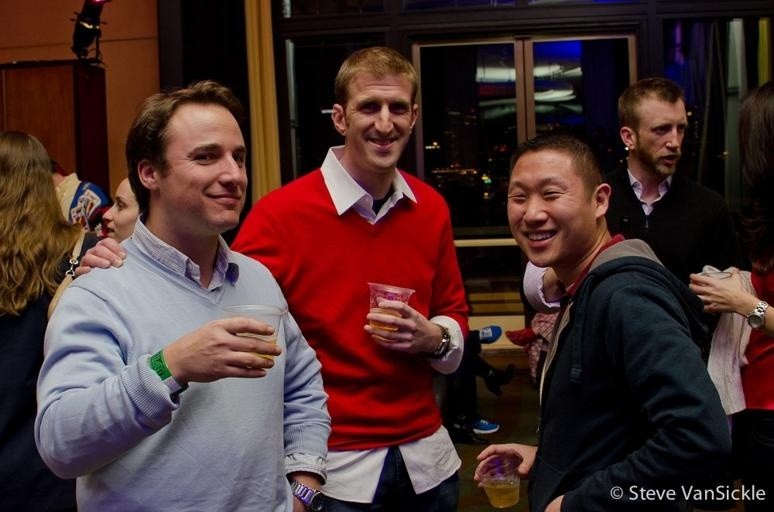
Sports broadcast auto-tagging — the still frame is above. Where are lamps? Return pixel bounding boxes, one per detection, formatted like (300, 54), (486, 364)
(68, 0), (113, 78)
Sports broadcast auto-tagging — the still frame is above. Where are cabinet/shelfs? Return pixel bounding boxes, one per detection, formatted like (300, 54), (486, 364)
(0, 65), (109, 192)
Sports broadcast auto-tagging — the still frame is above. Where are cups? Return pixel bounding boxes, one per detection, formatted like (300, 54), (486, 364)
(695, 271), (735, 284)
(219, 304), (289, 373)
(366, 281), (416, 340)
(474, 452), (525, 510)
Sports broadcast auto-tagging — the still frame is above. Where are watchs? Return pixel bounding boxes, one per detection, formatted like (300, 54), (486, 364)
(427, 323), (451, 358)
(289, 477), (327, 512)
(746, 300), (769, 330)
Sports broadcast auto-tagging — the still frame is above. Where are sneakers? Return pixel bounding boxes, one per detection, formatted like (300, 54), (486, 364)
(454, 412), (499, 435)
(478, 326), (501, 343)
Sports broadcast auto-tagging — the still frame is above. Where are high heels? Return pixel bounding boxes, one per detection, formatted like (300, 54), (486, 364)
(485, 364), (515, 397)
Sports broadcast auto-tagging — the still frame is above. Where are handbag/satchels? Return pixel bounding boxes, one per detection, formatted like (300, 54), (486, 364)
(47, 275), (74, 320)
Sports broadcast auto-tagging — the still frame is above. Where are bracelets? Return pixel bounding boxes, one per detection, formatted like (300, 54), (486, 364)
(148, 348), (188, 399)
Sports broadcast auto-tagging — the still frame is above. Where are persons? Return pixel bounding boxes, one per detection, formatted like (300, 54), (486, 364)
(468, 325), (501, 434)
(0, 128), (106, 512)
(33, 78), (334, 512)
(688, 77), (773, 511)
(72, 44), (469, 511)
(48, 158), (112, 225)
(441, 350), (514, 445)
(472, 132), (732, 511)
(102, 176), (143, 245)
(515, 76), (754, 316)
